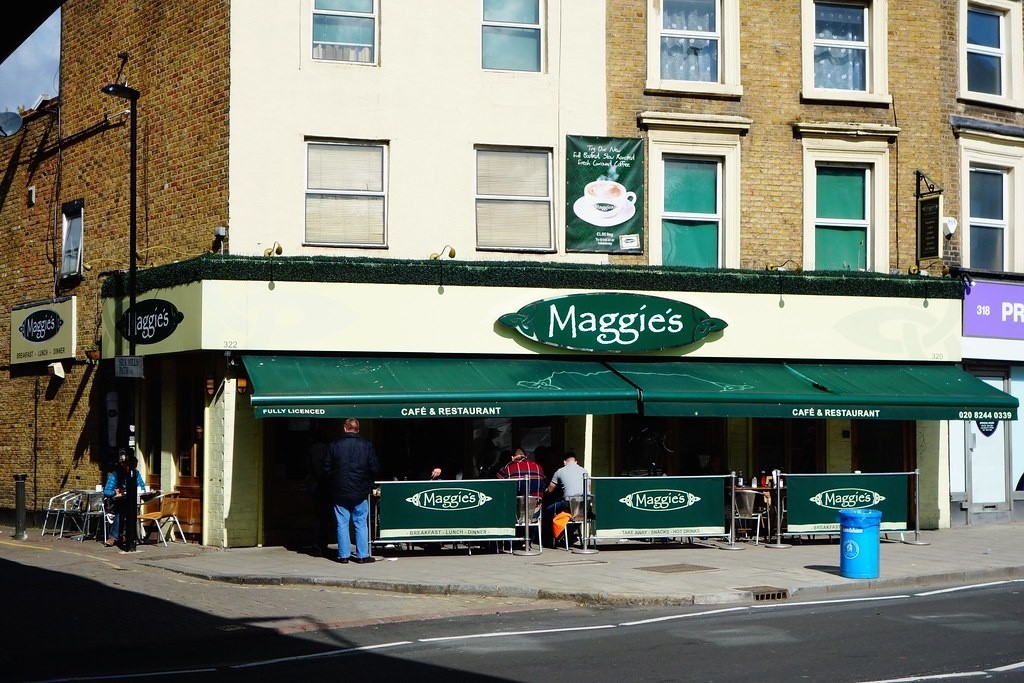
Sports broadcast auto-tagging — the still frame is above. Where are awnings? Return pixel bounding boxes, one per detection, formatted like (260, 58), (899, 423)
(607, 360), (1020, 422)
(240, 354), (639, 418)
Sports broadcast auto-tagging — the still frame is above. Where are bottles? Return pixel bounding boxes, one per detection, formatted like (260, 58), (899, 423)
(779, 475), (785, 487)
(738, 470), (743, 486)
(751, 476), (757, 488)
(760, 471), (766, 486)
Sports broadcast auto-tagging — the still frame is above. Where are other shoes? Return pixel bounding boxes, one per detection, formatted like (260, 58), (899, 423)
(106, 539), (114, 546)
(557, 535), (578, 547)
(522, 541), (532, 550)
(122, 536), (127, 544)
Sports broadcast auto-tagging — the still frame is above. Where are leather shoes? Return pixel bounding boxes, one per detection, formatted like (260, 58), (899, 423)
(357, 555), (375, 563)
(336, 556), (349, 563)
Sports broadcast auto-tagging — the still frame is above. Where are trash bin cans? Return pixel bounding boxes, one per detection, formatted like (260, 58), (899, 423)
(837, 507), (882, 579)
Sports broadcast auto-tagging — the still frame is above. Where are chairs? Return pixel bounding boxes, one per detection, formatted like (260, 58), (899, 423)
(553, 494), (597, 550)
(80, 496), (110, 544)
(729, 490), (832, 546)
(512, 495), (546, 557)
(137, 491), (187, 547)
(42, 490), (83, 538)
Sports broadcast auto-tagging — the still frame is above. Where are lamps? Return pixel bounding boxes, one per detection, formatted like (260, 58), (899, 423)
(909, 259), (950, 275)
(766, 260), (803, 273)
(264, 241), (283, 256)
(431, 245), (455, 260)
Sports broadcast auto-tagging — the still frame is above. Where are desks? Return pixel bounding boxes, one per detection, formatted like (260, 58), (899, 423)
(69, 489), (104, 540)
(733, 485), (786, 542)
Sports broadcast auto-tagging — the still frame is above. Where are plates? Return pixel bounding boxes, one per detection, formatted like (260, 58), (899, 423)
(573, 197), (635, 227)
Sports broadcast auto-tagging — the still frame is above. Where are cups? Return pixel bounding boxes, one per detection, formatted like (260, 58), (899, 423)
(137, 486), (141, 493)
(144, 486), (150, 492)
(585, 181), (636, 217)
(96, 485), (102, 492)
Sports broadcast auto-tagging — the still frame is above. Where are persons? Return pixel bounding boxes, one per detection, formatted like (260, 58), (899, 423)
(547, 452), (589, 543)
(104, 456), (146, 546)
(398, 418), (449, 481)
(326, 419), (379, 563)
(494, 449), (547, 521)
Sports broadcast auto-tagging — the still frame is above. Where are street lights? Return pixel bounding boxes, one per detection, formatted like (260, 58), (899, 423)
(99, 82), (141, 552)
